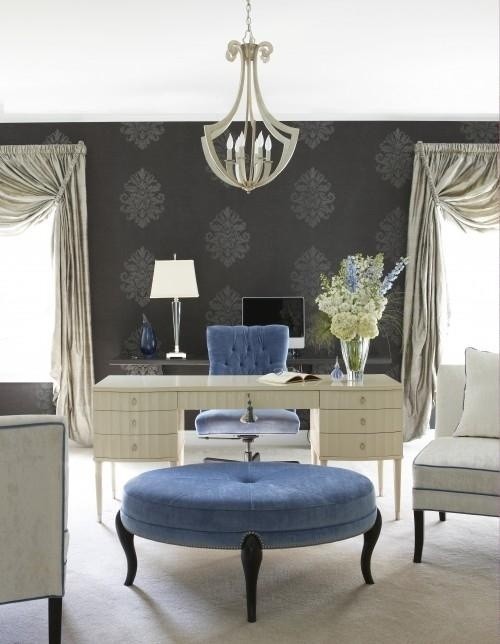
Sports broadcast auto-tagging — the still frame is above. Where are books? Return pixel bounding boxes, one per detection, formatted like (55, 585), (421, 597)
(257, 371), (326, 385)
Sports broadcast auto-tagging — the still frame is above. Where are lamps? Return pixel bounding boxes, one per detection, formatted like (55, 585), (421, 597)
(200, 0), (300, 192)
(149, 253), (201, 359)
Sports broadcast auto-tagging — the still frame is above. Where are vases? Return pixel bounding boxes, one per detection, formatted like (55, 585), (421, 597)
(340, 335), (370, 381)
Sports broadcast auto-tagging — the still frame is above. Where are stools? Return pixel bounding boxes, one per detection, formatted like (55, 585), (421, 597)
(115, 463), (382, 623)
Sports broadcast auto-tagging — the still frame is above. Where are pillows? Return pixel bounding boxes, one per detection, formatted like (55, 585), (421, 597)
(452, 347), (499, 439)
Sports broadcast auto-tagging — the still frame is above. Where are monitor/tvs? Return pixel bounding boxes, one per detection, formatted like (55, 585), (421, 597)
(241, 296), (305, 349)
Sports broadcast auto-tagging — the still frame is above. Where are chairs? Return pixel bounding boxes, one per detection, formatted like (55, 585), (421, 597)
(0, 414), (68, 644)
(195, 324), (300, 464)
(412, 364), (500, 563)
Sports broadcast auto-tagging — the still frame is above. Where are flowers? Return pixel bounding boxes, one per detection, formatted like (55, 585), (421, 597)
(314, 252), (410, 341)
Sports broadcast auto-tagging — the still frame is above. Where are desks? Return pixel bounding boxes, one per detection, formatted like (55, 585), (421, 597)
(93, 375), (404, 524)
(110, 358), (393, 375)
